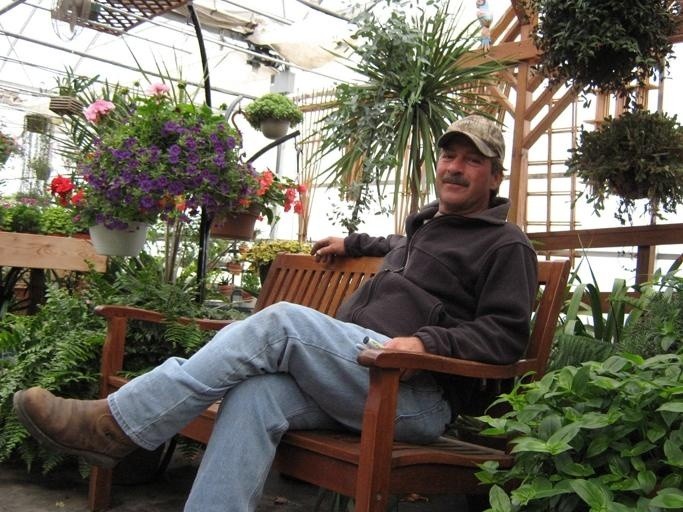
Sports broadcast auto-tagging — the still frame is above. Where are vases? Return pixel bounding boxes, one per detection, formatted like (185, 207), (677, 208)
(89, 219), (147, 256)
(210, 212), (258, 240)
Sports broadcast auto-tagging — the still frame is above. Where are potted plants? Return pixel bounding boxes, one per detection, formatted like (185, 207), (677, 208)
(524, 0), (682, 109)
(17, 205), (39, 234)
(239, 240), (249, 255)
(39, 206), (66, 237)
(218, 275), (235, 296)
(254, 240), (311, 287)
(0, 132), (26, 170)
(0, 204), (26, 232)
(476, 232), (683, 511)
(22, 112), (51, 132)
(65, 209), (90, 239)
(29, 157), (51, 180)
(0, 243), (310, 490)
(244, 92), (305, 139)
(226, 256), (242, 275)
(48, 64), (100, 116)
(299, 0), (523, 215)
(563, 109), (683, 226)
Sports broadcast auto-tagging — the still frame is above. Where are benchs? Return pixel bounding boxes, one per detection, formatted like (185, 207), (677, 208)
(90, 253), (571, 512)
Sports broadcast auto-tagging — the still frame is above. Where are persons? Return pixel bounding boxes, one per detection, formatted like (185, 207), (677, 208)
(12, 116), (539, 511)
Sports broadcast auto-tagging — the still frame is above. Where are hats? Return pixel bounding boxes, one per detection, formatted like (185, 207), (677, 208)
(438, 115), (505, 161)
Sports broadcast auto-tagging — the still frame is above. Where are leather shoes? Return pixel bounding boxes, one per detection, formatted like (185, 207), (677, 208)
(13, 386), (139, 470)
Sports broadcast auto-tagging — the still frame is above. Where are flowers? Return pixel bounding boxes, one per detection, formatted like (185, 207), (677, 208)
(47, 32), (261, 222)
(212, 164), (308, 224)
